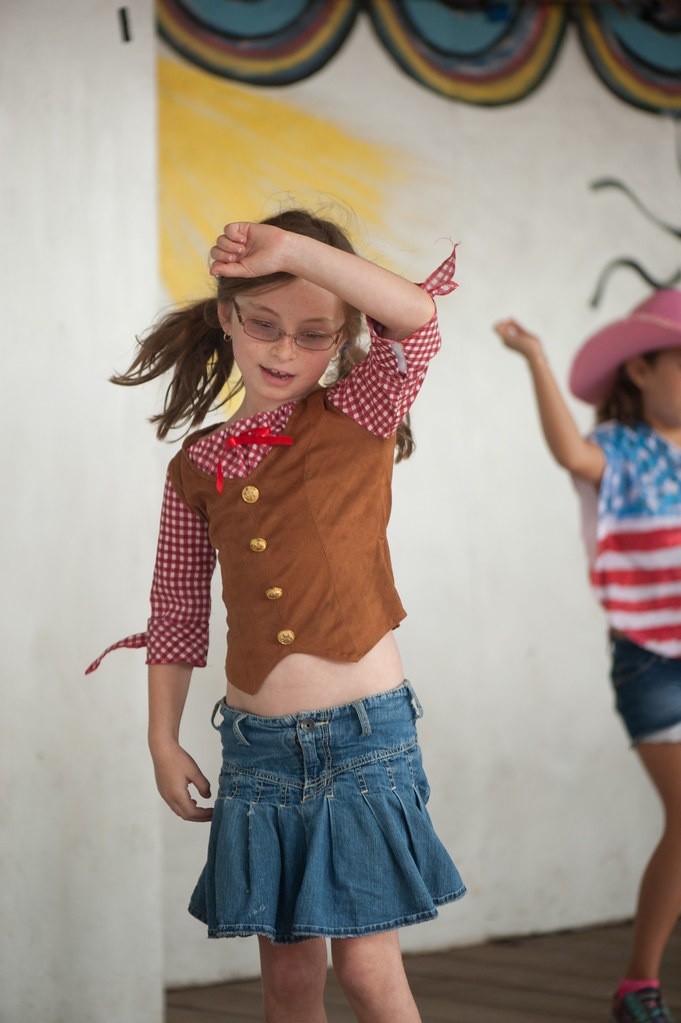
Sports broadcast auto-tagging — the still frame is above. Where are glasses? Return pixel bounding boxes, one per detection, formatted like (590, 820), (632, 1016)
(232, 297), (344, 351)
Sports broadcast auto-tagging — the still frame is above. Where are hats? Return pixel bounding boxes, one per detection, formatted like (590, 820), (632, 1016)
(569, 288), (681, 405)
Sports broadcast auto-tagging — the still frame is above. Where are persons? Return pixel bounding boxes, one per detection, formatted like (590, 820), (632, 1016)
(495, 290), (681, 1023)
(84, 208), (468, 1023)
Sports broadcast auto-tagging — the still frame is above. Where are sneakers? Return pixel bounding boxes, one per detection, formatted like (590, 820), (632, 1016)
(611, 978), (674, 1023)
